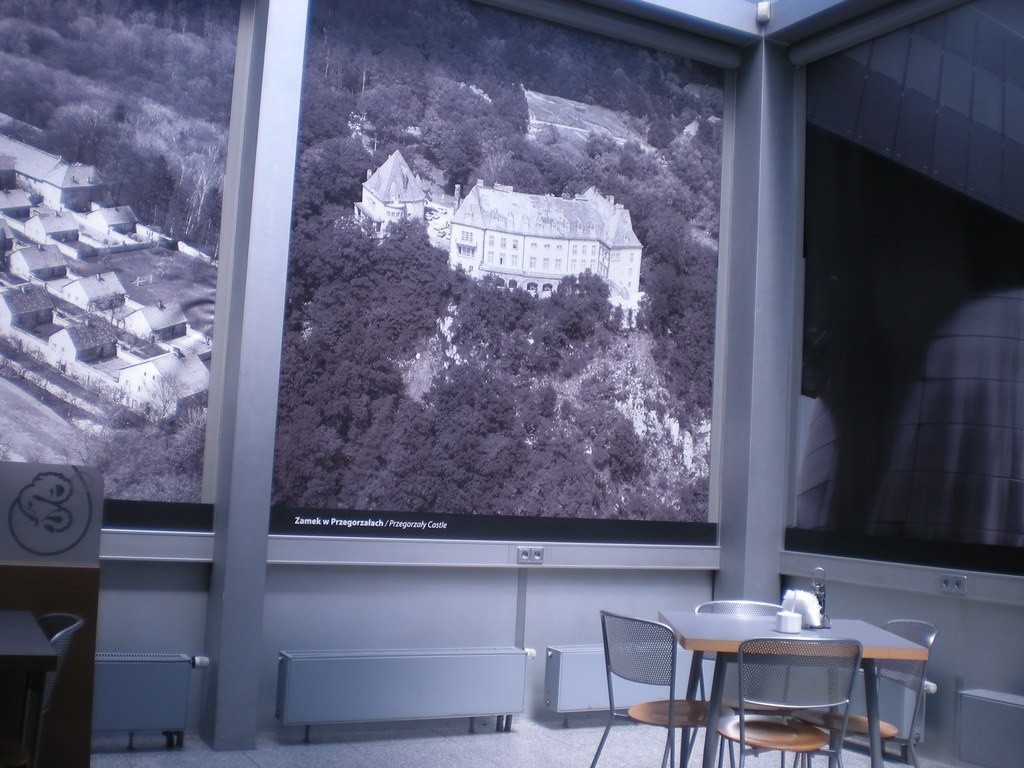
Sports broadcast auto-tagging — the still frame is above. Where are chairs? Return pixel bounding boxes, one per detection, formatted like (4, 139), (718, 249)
(715, 635), (863, 768)
(0, 612), (84, 768)
(791, 618), (939, 768)
(695, 599), (809, 768)
(588, 609), (736, 768)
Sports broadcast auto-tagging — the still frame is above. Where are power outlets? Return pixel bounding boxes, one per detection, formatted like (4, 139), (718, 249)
(517, 546), (544, 564)
(941, 573), (967, 595)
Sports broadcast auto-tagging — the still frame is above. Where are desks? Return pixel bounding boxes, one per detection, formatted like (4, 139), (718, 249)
(656, 611), (929, 768)
(0, 610), (58, 768)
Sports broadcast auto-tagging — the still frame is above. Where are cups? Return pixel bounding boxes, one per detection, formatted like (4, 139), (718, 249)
(776, 612), (802, 633)
